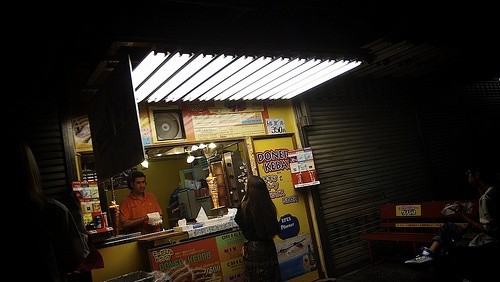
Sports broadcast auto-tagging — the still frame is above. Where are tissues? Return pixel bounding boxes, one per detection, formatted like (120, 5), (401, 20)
(173, 218), (193, 233)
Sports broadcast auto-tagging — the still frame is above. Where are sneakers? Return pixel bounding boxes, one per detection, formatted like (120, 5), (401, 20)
(405, 246), (437, 266)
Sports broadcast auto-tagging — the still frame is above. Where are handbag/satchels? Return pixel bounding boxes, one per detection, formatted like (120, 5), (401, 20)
(242, 240), (250, 262)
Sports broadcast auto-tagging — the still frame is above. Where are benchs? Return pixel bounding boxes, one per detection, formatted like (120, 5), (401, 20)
(364, 201), (476, 262)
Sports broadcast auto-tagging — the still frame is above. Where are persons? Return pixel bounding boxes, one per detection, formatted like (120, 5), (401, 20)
(404, 164), (500, 268)
(234, 175), (282, 282)
(119, 171), (163, 234)
(0, 137), (90, 282)
